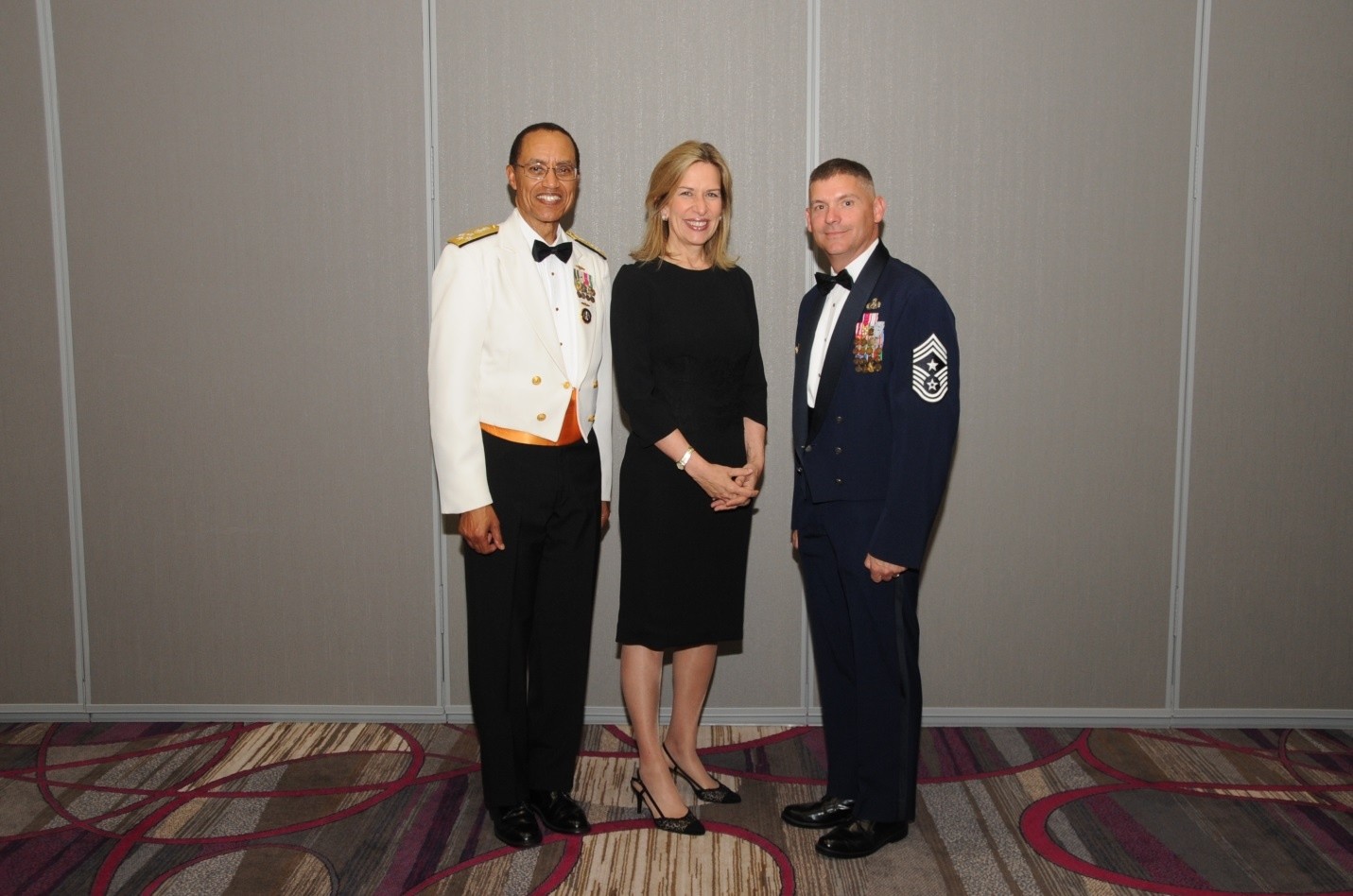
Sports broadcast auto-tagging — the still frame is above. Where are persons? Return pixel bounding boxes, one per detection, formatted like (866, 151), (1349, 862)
(608, 139), (769, 834)
(782, 155), (961, 861)
(427, 122), (614, 851)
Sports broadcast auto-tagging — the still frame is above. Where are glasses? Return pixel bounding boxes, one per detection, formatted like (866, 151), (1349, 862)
(512, 162), (579, 181)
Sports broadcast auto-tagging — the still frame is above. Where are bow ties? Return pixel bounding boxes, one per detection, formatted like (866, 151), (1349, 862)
(815, 269), (854, 294)
(532, 239), (573, 264)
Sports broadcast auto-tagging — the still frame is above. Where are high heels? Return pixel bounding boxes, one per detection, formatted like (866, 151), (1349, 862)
(630, 769), (706, 836)
(673, 765), (742, 804)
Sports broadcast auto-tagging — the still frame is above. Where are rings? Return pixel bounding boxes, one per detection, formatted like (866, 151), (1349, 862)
(892, 574), (900, 578)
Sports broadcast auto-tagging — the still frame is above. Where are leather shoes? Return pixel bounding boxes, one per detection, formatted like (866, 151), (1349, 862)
(529, 789), (590, 835)
(488, 802), (543, 849)
(781, 792), (863, 830)
(814, 814), (909, 859)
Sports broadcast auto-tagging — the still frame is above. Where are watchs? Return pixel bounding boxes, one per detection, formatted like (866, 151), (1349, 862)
(675, 446), (695, 470)
